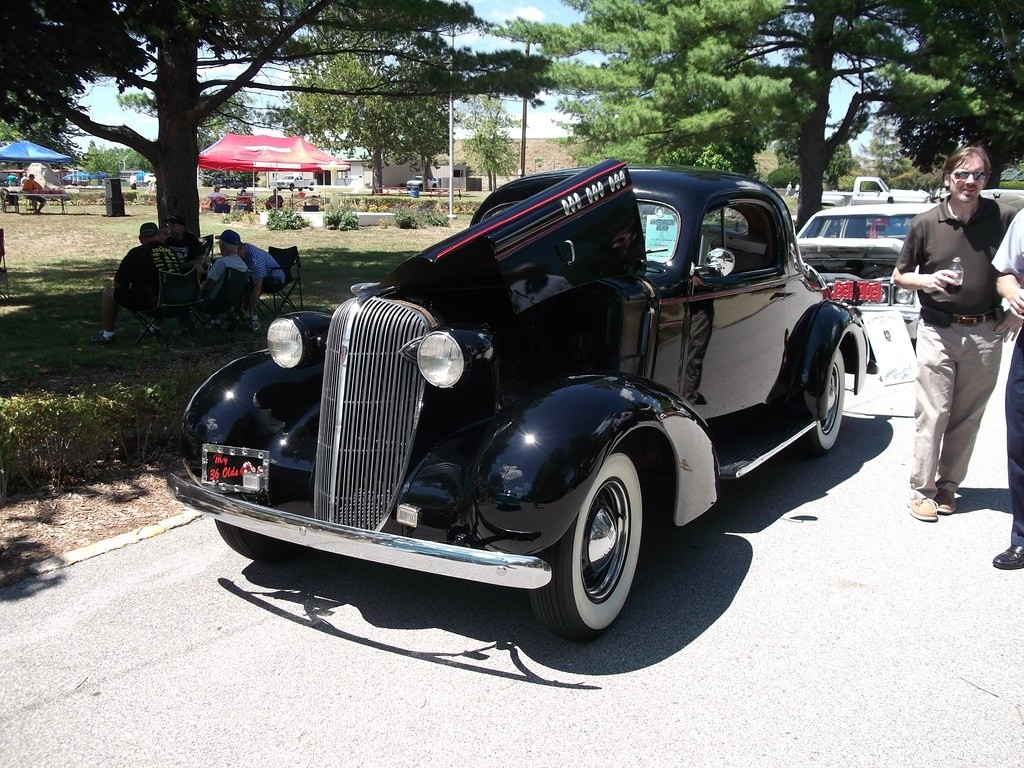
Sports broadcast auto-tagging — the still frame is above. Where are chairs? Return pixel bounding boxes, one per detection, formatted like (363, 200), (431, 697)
(0, 228), (10, 305)
(255, 247), (305, 321)
(3, 188), (20, 213)
(237, 191), (254, 212)
(194, 268), (256, 341)
(185, 231), (213, 271)
(122, 268), (201, 350)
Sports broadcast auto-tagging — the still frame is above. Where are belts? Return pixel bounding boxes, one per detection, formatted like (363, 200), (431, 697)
(952, 311), (995, 326)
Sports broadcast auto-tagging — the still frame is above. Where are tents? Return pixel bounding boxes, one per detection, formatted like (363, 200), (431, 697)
(0, 140), (72, 215)
(199, 132), (348, 212)
(134, 171), (146, 180)
(63, 170), (111, 181)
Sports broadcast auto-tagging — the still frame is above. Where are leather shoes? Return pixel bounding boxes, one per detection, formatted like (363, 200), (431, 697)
(992, 545), (1024, 570)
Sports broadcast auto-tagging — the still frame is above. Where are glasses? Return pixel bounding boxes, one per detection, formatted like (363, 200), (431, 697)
(949, 171), (989, 180)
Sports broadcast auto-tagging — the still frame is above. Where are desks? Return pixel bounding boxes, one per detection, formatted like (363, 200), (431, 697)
(9, 192), (68, 215)
(425, 188), (463, 193)
(376, 186), (410, 192)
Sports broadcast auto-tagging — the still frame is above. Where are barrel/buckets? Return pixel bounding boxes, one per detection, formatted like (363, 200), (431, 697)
(410, 187), (419, 198)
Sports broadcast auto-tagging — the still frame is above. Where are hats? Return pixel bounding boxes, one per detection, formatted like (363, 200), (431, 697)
(164, 215), (185, 226)
(140, 223), (159, 236)
(216, 230), (240, 245)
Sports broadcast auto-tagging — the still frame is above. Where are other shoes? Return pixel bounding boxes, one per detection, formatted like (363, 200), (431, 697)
(174, 326), (197, 337)
(89, 332), (116, 345)
(249, 315), (260, 332)
(212, 322), (224, 333)
(141, 326), (162, 338)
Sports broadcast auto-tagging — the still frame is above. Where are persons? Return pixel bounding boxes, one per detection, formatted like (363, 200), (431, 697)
(607, 224), (637, 257)
(159, 218), (205, 280)
(679, 285), (713, 407)
(22, 174), (46, 214)
(208, 185), (230, 213)
(88, 222), (182, 346)
(266, 188), (283, 210)
(178, 229), (248, 329)
(237, 190), (254, 213)
(992, 204), (1024, 570)
(511, 262), (573, 312)
(891, 146), (1019, 522)
(98, 180), (102, 186)
(236, 241), (285, 331)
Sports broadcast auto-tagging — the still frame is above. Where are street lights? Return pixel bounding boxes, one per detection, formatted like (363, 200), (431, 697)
(124, 156), (128, 171)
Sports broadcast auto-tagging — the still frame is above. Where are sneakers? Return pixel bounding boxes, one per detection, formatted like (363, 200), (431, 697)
(934, 488), (956, 514)
(908, 498), (938, 521)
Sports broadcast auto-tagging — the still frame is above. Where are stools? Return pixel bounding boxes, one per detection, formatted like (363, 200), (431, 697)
(26, 197), (36, 214)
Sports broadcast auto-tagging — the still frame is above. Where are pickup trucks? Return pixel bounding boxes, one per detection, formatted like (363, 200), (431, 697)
(406, 175), (438, 191)
(820, 175), (931, 207)
(277, 175), (317, 191)
(129, 173), (157, 187)
(212, 176), (250, 189)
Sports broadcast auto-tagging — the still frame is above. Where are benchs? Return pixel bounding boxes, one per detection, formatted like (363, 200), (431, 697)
(424, 194), (463, 198)
(371, 192), (410, 195)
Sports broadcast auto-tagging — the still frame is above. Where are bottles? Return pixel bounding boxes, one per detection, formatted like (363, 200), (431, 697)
(945, 257), (963, 295)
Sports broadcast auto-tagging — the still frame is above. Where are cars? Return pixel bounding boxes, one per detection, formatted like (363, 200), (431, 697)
(166, 163), (878, 642)
(795, 186), (1024, 339)
